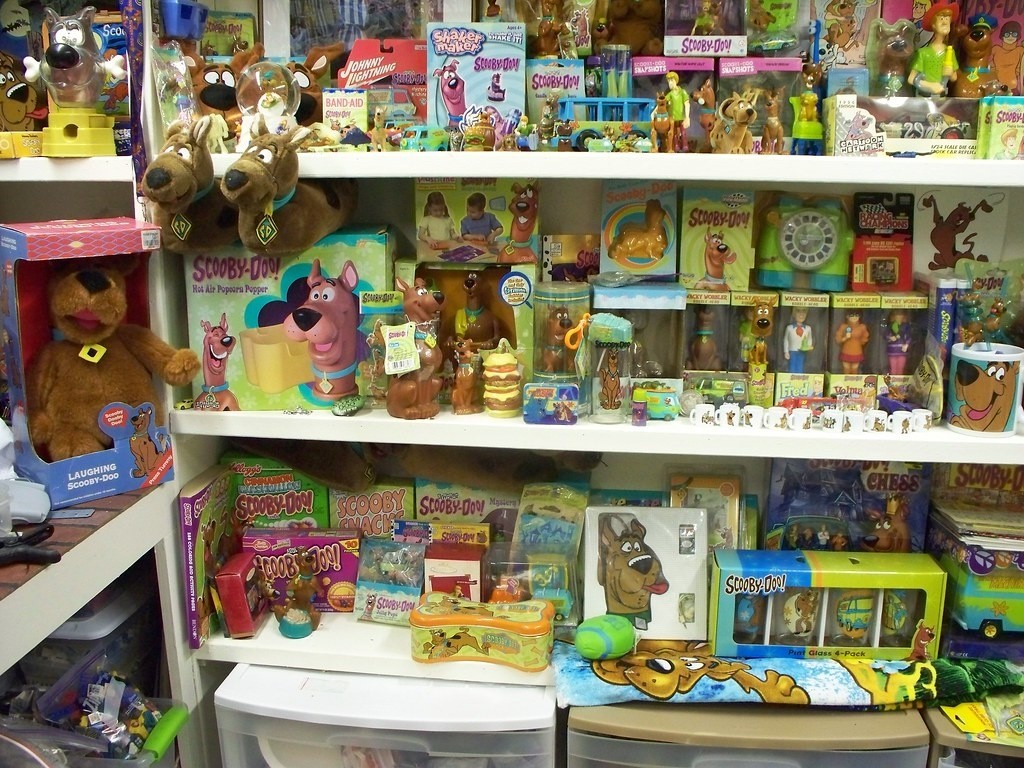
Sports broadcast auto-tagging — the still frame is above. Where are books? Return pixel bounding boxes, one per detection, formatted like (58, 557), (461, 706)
(472, 239), (498, 247)
(430, 241), (457, 250)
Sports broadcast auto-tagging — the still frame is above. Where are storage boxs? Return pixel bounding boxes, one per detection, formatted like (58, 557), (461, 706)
(388, 518), (492, 602)
(415, 470), (591, 542)
(329, 478), (416, 539)
(407, 590), (555, 669)
(707, 547), (950, 658)
(921, 707), (1024, 768)
(758, 459), (932, 556)
(68, 696), (190, 768)
(176, 176), (930, 439)
(579, 505), (710, 641)
(220, 448), (330, 530)
(239, 527), (364, 614)
(212, 663), (558, 768)
(155, 0), (1024, 160)
(0, 216), (176, 509)
(422, 541), (487, 601)
(213, 553), (271, 637)
(562, 702), (931, 768)
(17, 567), (161, 692)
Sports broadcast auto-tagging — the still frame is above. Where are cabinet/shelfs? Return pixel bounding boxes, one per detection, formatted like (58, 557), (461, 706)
(0, 157), (213, 768)
(133, 0), (1024, 768)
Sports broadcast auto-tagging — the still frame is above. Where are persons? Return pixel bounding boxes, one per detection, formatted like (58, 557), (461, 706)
(783, 306), (910, 375)
(418, 192), (463, 248)
(651, 71), (690, 153)
(908, 0), (959, 97)
(461, 192), (503, 244)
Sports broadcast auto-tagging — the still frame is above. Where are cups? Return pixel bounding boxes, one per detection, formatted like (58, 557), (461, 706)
(820, 409), (843, 432)
(947, 343), (1024, 438)
(740, 405), (763, 429)
(843, 411), (864, 433)
(787, 408), (812, 432)
(887, 411), (913, 434)
(764, 407), (788, 431)
(715, 404), (739, 428)
(690, 404), (715, 427)
(912, 409), (932, 432)
(864, 410), (887, 433)
(583, 322), (635, 424)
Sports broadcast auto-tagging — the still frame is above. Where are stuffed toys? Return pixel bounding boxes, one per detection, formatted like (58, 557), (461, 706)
(140, 43), (356, 258)
(24, 253), (201, 462)
